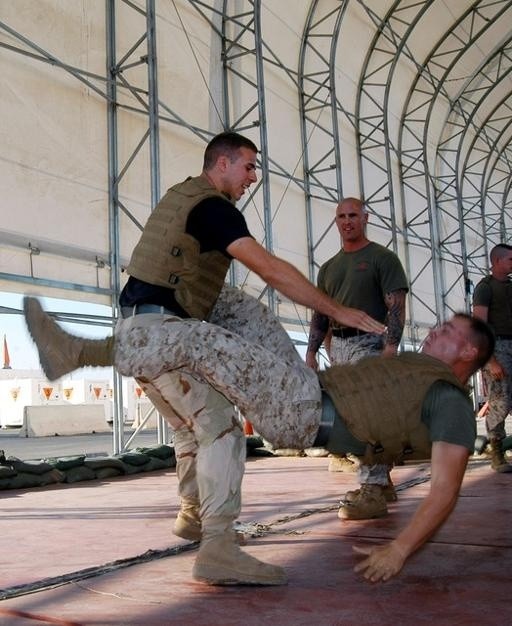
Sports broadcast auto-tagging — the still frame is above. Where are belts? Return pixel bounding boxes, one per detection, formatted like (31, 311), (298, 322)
(331, 328), (367, 340)
(310, 391), (338, 449)
(119, 300), (181, 317)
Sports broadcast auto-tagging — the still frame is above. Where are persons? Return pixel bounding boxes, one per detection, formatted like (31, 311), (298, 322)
(323, 449), (364, 474)
(303, 194), (410, 520)
(119, 128), (391, 586)
(21, 281), (498, 583)
(471, 241), (511, 472)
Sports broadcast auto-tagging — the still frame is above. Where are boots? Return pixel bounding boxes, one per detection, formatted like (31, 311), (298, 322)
(192, 531), (288, 587)
(491, 438), (512, 474)
(328, 454), (361, 472)
(338, 472), (399, 521)
(173, 492), (245, 545)
(22, 296), (117, 382)
